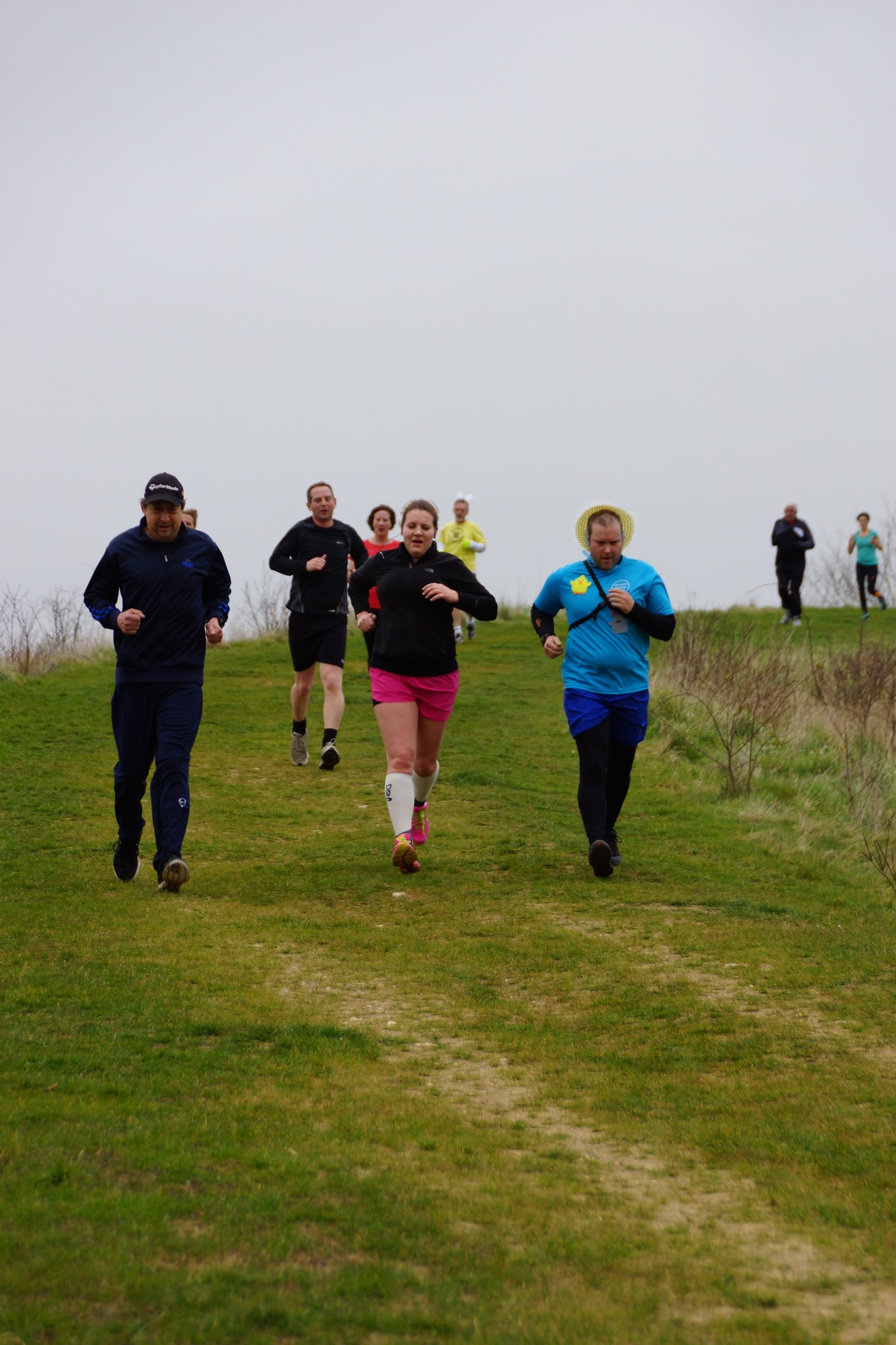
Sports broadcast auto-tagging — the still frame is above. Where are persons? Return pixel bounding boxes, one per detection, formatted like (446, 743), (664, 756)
(181, 508), (198, 528)
(439, 499), (487, 642)
(530, 504), (676, 878)
(268, 482), (371, 770)
(847, 513), (887, 619)
(83, 472), (231, 893)
(349, 505), (402, 667)
(772, 504), (814, 627)
(349, 500), (498, 876)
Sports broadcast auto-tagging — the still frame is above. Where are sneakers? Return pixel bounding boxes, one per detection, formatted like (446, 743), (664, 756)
(318, 738), (339, 769)
(454, 632), (464, 643)
(112, 835), (141, 882)
(290, 727), (309, 766)
(467, 622), (476, 639)
(588, 838), (614, 877)
(392, 833), (421, 874)
(608, 829), (622, 865)
(410, 802), (428, 844)
(156, 856), (189, 892)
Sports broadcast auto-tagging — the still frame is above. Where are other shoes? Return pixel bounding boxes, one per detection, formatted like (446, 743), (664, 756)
(793, 618), (802, 626)
(780, 612), (793, 623)
(877, 596), (886, 609)
(860, 614), (869, 620)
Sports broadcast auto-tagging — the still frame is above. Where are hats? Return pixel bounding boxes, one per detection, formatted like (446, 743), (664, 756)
(577, 505), (633, 552)
(144, 471), (185, 506)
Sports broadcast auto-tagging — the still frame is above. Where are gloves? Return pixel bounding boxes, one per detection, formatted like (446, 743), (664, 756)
(461, 539), (472, 549)
